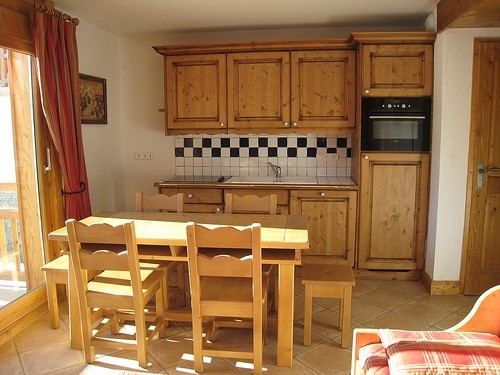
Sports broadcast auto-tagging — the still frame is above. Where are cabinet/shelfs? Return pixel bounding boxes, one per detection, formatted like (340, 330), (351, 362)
(150, 31), (434, 281)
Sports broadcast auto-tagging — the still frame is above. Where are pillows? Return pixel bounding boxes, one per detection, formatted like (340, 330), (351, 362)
(376, 329), (500, 375)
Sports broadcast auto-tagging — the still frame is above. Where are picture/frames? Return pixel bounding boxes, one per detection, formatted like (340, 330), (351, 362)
(79, 72), (108, 124)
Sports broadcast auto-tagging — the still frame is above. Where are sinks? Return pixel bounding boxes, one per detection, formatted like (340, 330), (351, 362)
(224, 176), (319, 184)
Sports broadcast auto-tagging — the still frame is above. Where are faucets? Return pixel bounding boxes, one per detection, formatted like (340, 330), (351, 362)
(266, 161), (281, 178)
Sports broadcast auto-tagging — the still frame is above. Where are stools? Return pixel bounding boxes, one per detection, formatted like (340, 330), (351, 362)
(302, 263), (355, 348)
(40, 255), (102, 329)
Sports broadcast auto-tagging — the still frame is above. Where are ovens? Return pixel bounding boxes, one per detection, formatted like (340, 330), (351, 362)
(360, 97), (431, 153)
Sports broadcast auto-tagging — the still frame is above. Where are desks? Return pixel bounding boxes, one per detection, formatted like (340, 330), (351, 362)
(48, 211), (310, 367)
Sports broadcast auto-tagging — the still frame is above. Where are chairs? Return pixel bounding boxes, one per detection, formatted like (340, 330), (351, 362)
(66, 193), (277, 375)
(351, 285), (500, 375)
